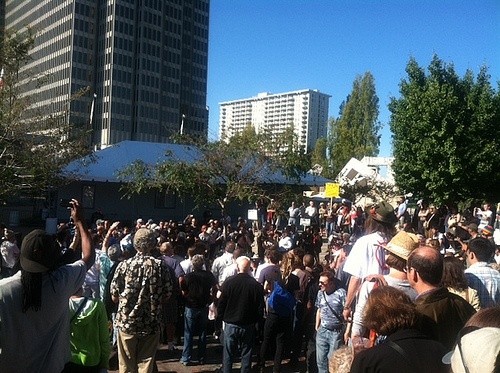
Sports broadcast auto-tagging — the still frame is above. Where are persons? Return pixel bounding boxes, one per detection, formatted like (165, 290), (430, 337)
(0, 197), (94, 373)
(0, 196), (500, 365)
(450, 324), (500, 373)
(314, 273), (350, 373)
(256, 256), (300, 373)
(70, 288), (111, 373)
(351, 286), (453, 373)
(108, 230), (177, 373)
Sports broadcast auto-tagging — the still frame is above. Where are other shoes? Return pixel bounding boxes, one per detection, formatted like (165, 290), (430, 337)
(179, 359), (189, 366)
(199, 360), (206, 365)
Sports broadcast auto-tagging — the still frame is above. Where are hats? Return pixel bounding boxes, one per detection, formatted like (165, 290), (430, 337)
(19, 229), (62, 273)
(468, 223), (478, 231)
(482, 225), (494, 232)
(377, 231), (418, 261)
(442, 326), (481, 366)
(367, 201), (399, 226)
(450, 327), (500, 373)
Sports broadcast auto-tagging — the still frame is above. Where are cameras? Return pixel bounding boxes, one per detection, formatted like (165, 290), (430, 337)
(60, 198), (74, 208)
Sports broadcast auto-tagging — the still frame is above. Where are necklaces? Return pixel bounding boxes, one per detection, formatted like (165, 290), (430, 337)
(214, 256), (266, 373)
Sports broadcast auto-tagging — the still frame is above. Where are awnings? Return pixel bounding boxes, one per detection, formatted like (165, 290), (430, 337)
(58, 138), (334, 186)
